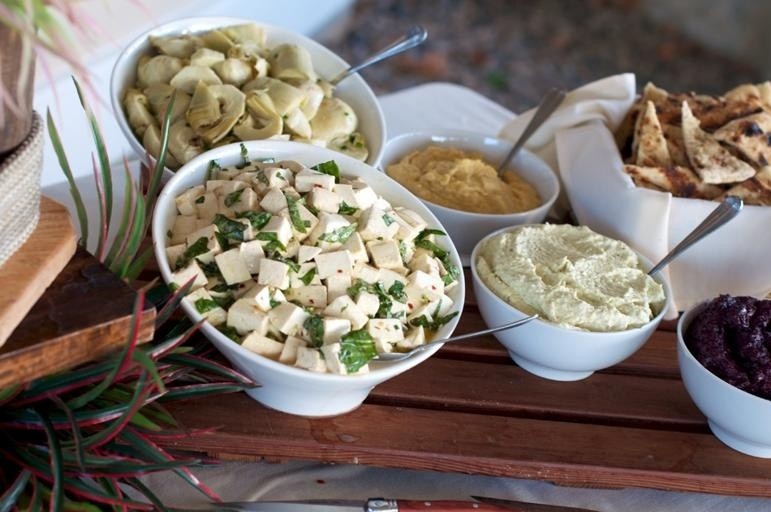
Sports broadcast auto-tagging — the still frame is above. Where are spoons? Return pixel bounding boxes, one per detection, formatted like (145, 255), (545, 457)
(368, 311), (540, 363)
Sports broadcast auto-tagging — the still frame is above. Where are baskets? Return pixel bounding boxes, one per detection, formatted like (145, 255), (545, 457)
(1, 110), (45, 267)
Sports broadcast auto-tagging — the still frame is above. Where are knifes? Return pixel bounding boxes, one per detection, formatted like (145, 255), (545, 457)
(206, 495), (597, 512)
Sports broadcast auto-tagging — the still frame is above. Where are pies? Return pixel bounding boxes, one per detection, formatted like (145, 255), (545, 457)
(616, 79), (771, 207)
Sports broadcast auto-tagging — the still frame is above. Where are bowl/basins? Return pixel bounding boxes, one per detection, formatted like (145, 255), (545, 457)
(109, 15), (389, 184)
(374, 133), (565, 267)
(150, 141), (469, 423)
(469, 221), (674, 386)
(676, 295), (770, 460)
